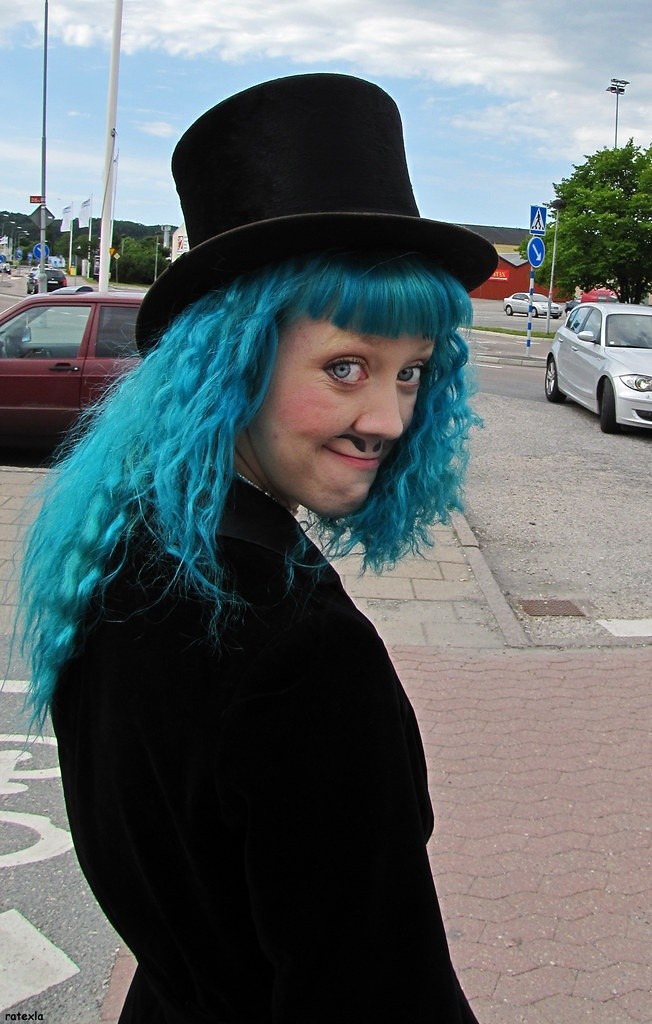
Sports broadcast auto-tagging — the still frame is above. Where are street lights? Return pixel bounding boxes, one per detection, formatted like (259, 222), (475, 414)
(121, 234), (127, 255)
(11, 226), (22, 251)
(9, 221), (16, 238)
(544, 200), (566, 334)
(16, 230), (28, 249)
(605, 78), (631, 149)
(154, 231), (163, 282)
(1, 214), (10, 239)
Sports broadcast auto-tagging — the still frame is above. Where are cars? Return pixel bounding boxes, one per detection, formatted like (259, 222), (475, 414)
(565, 288), (626, 310)
(0, 284), (149, 446)
(503, 293), (563, 319)
(26, 263), (68, 294)
(544, 301), (652, 435)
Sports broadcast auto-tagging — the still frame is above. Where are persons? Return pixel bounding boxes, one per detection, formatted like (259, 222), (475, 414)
(20, 73), (501, 1024)
(33, 279), (39, 294)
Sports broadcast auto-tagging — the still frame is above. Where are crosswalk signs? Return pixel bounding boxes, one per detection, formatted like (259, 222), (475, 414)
(529, 205), (547, 235)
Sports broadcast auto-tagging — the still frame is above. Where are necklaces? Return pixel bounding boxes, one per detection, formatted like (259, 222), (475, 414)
(234, 468), (297, 517)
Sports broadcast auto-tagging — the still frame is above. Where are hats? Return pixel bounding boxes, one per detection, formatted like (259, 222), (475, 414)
(135, 73), (499, 360)
